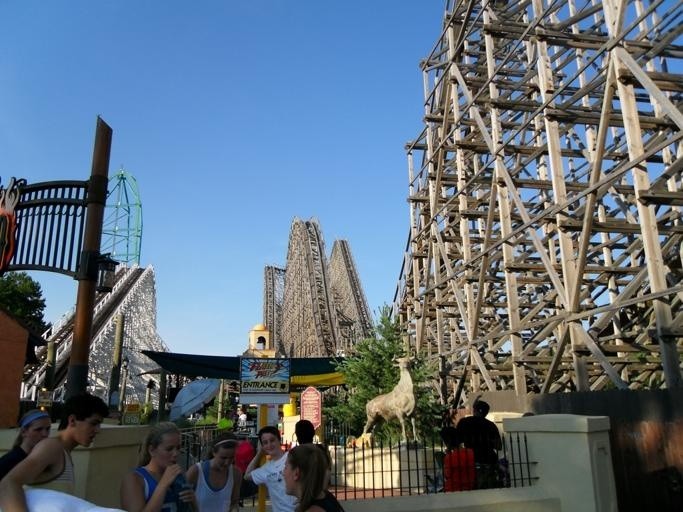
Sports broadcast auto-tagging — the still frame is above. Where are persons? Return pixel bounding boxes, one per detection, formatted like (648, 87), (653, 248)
(293, 417), (333, 492)
(241, 424), (299, 511)
(117, 420), (196, 511)
(0, 409), (52, 477)
(181, 431), (242, 511)
(422, 397), (536, 493)
(233, 406), (247, 432)
(281, 441), (345, 511)
(0, 391), (109, 512)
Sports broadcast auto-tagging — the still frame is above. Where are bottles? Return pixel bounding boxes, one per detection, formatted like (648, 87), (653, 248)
(172, 473), (189, 512)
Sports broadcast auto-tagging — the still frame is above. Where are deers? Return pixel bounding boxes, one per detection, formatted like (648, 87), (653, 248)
(362, 347), (423, 449)
